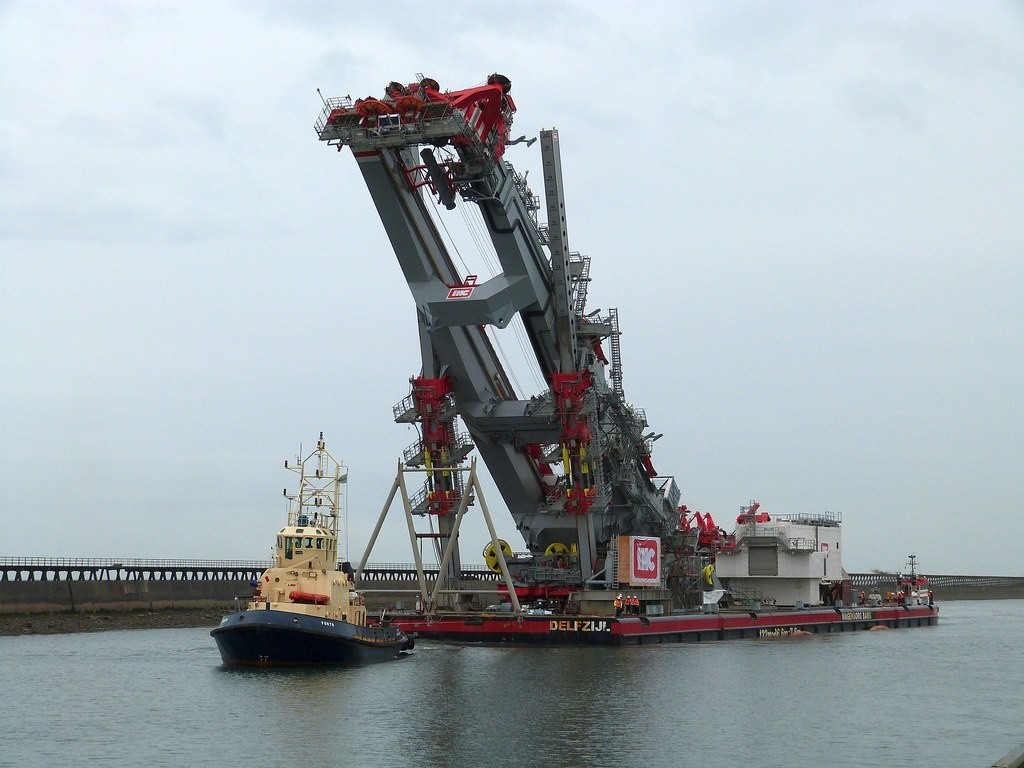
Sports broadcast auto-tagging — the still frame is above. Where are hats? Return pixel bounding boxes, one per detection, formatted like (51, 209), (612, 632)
(627, 596), (630, 598)
(634, 596), (637, 598)
(617, 593), (622, 599)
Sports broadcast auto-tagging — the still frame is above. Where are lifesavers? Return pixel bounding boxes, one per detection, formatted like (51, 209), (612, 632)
(359, 592), (363, 604)
(406, 633), (414, 651)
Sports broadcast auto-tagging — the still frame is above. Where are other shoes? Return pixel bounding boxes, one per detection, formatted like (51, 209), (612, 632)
(632, 614), (636, 615)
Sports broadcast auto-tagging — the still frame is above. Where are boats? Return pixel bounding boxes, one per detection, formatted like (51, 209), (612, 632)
(208, 429), (415, 668)
(312, 72), (941, 644)
(289, 590), (329, 605)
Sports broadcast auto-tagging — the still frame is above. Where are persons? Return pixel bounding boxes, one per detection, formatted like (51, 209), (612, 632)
(899, 590), (904, 605)
(614, 593), (639, 619)
(860, 591), (865, 604)
